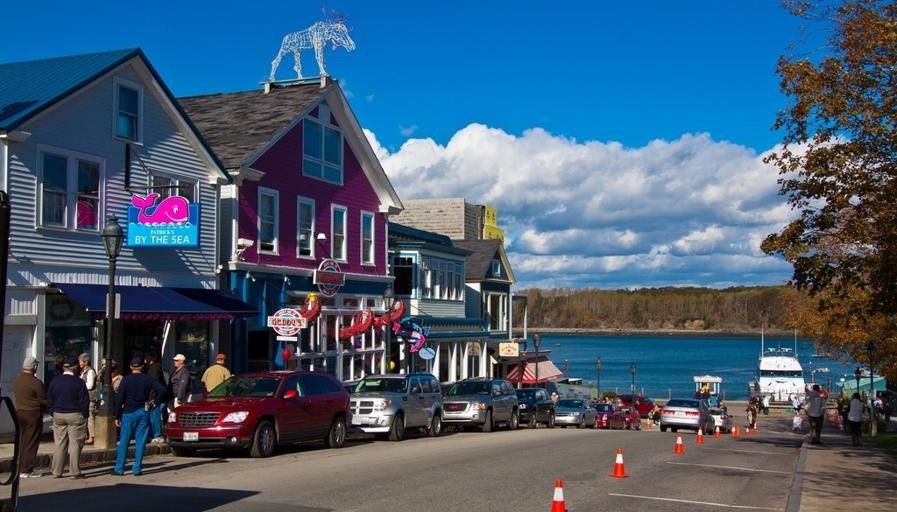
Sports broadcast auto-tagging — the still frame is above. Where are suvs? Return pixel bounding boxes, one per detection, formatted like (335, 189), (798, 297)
(164, 370), (352, 457)
(618, 393), (662, 419)
(348, 372), (444, 442)
(441, 376), (520, 433)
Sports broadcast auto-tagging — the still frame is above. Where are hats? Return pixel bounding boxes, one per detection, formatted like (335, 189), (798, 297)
(23, 357), (39, 369)
(130, 356), (144, 367)
(172, 354), (184, 361)
(62, 358), (77, 367)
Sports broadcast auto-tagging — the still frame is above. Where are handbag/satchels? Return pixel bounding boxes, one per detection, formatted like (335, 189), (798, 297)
(704, 415), (715, 434)
(793, 414), (810, 435)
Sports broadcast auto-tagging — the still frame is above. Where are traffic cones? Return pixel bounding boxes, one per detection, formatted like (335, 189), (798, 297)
(730, 422), (758, 438)
(713, 425), (720, 439)
(550, 480), (567, 512)
(671, 431), (685, 454)
(694, 425), (705, 443)
(607, 448), (629, 477)
(645, 418), (651, 430)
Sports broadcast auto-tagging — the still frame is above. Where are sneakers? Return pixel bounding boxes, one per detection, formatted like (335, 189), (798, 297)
(149, 436), (164, 443)
(20, 472), (44, 478)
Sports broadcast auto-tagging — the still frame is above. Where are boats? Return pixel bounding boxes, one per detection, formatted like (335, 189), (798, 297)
(748, 322), (807, 403)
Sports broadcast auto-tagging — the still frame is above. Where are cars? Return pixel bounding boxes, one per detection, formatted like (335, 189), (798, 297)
(706, 406), (732, 432)
(552, 397), (598, 429)
(616, 404), (641, 430)
(658, 398), (715, 434)
(590, 402), (625, 430)
(515, 387), (555, 429)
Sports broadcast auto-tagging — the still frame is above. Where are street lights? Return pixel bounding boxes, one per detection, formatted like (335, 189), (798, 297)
(382, 284), (395, 374)
(854, 367), (862, 394)
(594, 356), (602, 398)
(532, 327), (541, 387)
(92, 213), (126, 446)
(630, 363), (637, 402)
(866, 340), (877, 389)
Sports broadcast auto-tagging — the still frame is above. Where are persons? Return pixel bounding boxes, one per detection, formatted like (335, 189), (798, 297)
(98, 356), (166, 446)
(747, 391), (777, 415)
(201, 355), (231, 394)
(695, 383), (726, 434)
(11, 358), (43, 478)
(48, 357), (89, 480)
(167, 353), (190, 408)
(110, 356), (164, 475)
(592, 395), (661, 428)
(550, 391), (558, 405)
(78, 352), (98, 444)
(791, 383), (883, 446)
